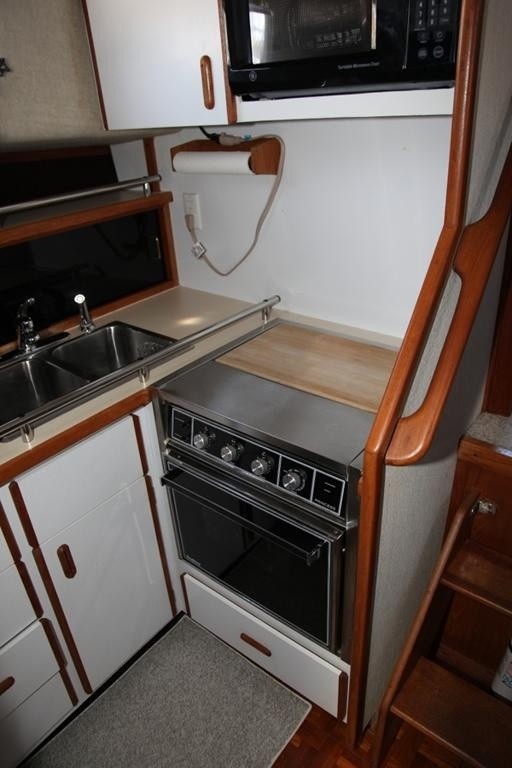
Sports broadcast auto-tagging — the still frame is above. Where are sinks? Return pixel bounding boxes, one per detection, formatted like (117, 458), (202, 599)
(0, 350), (92, 442)
(35, 321), (195, 383)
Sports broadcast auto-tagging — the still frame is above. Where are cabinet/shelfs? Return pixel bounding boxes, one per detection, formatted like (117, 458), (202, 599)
(81, 0), (511, 133)
(0, 282), (270, 764)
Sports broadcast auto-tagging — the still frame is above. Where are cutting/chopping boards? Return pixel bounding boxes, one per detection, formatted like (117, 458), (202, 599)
(215, 324), (397, 415)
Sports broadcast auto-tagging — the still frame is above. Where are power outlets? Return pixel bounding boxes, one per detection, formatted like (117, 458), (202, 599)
(181, 193), (204, 235)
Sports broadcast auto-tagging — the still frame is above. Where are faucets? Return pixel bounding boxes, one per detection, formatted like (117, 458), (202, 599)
(15, 297), (41, 352)
(74, 294), (96, 332)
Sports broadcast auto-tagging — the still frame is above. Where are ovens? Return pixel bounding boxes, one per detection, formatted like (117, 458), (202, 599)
(152, 401), (362, 664)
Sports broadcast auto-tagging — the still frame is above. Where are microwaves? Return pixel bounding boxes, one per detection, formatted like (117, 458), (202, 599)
(220, 0), (465, 101)
